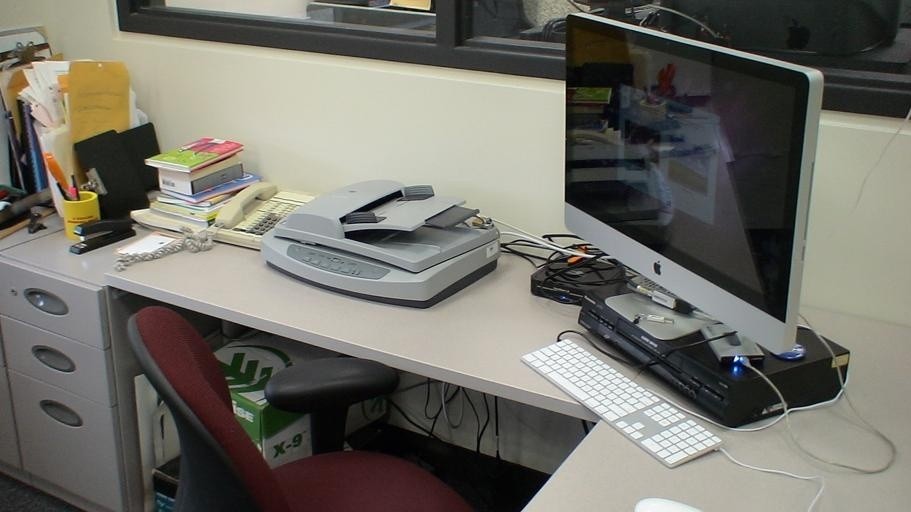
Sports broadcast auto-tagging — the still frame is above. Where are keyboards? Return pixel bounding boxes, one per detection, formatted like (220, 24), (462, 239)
(519, 337), (724, 470)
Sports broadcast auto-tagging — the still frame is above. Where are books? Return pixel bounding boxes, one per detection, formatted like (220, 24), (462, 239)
(0, 96), (264, 239)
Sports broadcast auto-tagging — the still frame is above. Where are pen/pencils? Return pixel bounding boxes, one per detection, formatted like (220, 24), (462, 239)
(44, 152), (80, 201)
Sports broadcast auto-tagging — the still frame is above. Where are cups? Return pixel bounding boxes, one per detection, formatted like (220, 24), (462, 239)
(62, 191), (101, 241)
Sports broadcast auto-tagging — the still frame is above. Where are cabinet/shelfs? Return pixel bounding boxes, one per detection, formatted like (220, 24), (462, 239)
(0, 208), (67, 488)
(0, 206), (224, 512)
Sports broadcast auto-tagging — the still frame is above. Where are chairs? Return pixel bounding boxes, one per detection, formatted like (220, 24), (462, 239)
(122, 304), (472, 512)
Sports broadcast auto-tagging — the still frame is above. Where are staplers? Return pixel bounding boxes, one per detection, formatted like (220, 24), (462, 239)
(70, 216), (136, 255)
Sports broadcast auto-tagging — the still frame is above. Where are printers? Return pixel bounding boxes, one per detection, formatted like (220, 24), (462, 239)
(261, 180), (501, 308)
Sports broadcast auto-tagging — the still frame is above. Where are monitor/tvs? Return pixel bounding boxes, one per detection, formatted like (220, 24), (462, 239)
(564, 12), (825, 353)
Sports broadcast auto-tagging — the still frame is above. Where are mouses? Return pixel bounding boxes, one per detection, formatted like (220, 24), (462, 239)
(635, 497), (698, 512)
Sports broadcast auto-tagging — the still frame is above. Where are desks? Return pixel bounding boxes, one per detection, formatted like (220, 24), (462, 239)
(104, 199), (911, 512)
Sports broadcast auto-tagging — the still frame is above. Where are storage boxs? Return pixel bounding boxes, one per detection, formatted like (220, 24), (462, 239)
(204, 330), (392, 472)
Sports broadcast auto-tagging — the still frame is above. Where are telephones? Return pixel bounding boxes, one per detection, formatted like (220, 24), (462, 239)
(206, 181), (316, 250)
